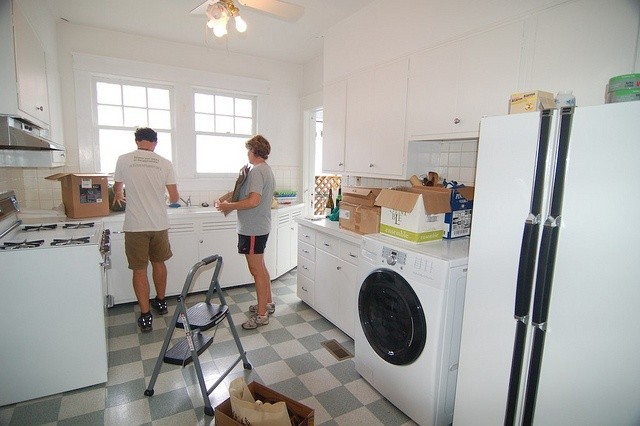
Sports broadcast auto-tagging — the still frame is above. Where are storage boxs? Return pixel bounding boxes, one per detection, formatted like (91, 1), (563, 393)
(414, 182), (474, 239)
(341, 186), (381, 209)
(338, 200), (381, 237)
(44, 172), (113, 219)
(215, 381), (314, 426)
(373, 186), (450, 245)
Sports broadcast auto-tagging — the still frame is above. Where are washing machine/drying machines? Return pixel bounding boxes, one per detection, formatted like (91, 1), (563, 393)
(355, 234), (470, 426)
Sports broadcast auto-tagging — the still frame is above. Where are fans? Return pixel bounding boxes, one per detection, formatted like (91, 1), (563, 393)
(186, 0), (303, 22)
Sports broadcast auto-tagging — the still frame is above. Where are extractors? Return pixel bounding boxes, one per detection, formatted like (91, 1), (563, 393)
(0, 116), (68, 169)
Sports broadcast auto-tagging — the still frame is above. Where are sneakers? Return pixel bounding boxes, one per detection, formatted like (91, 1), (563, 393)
(249, 304), (275, 313)
(137, 313), (152, 333)
(241, 312), (269, 330)
(150, 296), (168, 315)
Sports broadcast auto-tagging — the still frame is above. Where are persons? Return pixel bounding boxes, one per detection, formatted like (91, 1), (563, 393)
(216, 133), (277, 330)
(112, 127), (179, 333)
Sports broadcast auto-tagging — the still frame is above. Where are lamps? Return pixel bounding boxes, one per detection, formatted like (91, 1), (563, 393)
(201, 0), (247, 36)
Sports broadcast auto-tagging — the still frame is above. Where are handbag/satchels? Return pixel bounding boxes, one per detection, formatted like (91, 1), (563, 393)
(232, 164), (251, 202)
(229, 375), (292, 426)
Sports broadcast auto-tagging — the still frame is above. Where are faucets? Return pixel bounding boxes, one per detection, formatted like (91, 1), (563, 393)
(186, 194), (192, 207)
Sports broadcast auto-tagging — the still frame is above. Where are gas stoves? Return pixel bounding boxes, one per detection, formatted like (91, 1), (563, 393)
(0, 221), (103, 253)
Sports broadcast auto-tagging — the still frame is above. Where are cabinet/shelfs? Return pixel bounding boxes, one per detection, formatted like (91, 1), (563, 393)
(343, 55), (411, 180)
(317, 231), (362, 339)
(519, 3), (640, 107)
(296, 224), (317, 310)
(106, 223), (149, 306)
(275, 202), (307, 277)
(1, 228), (115, 406)
(155, 209), (278, 300)
(406, 16), (525, 142)
(322, 80), (346, 176)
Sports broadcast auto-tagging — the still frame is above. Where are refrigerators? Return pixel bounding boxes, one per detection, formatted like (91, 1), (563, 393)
(451, 98), (639, 425)
